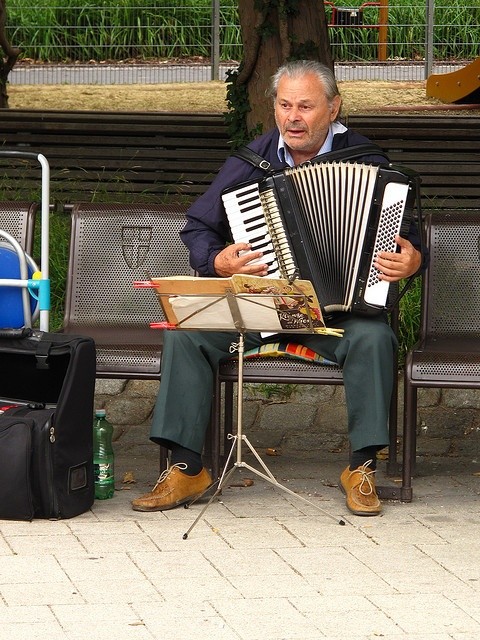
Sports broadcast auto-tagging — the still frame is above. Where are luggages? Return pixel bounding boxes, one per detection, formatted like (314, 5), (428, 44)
(0, 326), (96, 523)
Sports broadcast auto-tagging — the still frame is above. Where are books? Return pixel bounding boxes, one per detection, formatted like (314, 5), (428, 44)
(148, 273), (326, 333)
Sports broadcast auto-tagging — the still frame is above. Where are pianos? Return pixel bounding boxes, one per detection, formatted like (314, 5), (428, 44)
(220, 159), (418, 343)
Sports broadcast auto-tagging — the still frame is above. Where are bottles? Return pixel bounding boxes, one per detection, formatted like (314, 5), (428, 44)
(93, 409), (117, 500)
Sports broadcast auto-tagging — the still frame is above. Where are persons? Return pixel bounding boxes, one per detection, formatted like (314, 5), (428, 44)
(130, 59), (432, 516)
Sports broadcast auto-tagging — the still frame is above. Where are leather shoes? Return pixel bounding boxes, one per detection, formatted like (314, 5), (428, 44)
(338, 459), (382, 516)
(131, 458), (215, 512)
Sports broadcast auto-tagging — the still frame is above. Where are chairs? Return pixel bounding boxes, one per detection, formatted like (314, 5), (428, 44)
(212, 219), (400, 499)
(63, 201), (198, 477)
(1, 202), (36, 252)
(400, 208), (480, 502)
